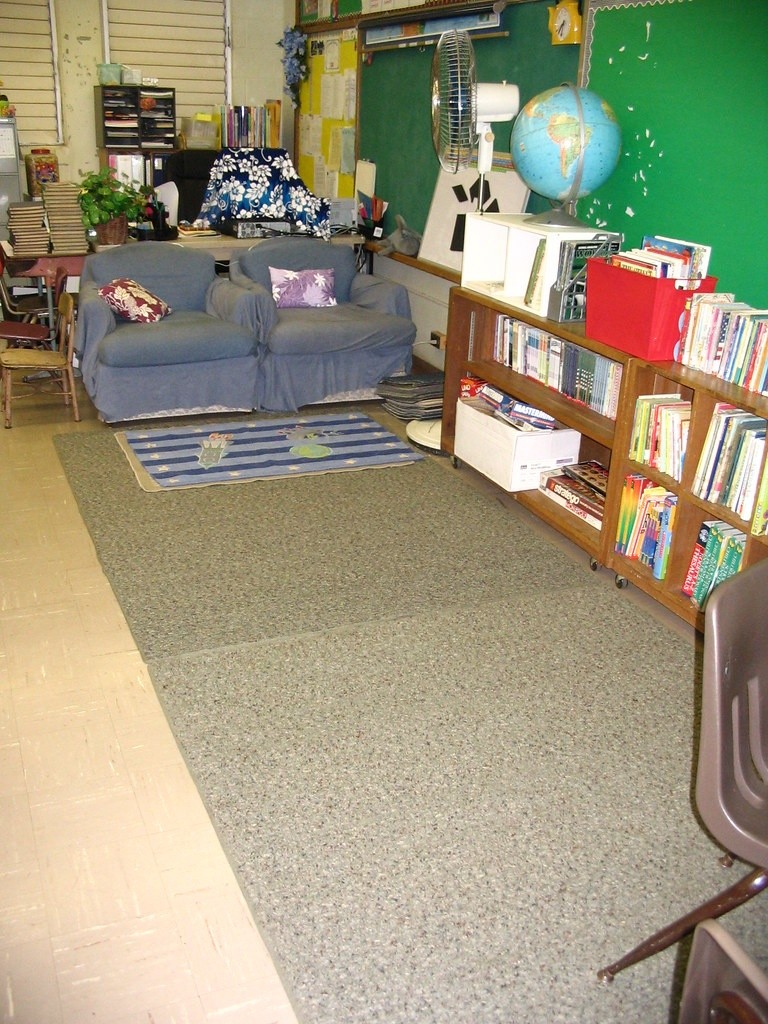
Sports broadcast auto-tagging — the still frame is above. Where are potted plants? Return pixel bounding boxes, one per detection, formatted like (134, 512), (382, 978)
(78, 167), (157, 245)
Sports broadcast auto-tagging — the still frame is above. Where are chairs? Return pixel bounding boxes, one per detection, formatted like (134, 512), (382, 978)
(598, 559), (768, 982)
(0, 259), (81, 429)
(164, 150), (218, 225)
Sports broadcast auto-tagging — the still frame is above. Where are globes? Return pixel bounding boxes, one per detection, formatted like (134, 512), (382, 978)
(510, 80), (623, 230)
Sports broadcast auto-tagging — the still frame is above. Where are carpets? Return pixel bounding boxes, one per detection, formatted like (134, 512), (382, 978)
(125, 412), (424, 487)
(114, 427), (415, 492)
(51, 406), (768, 1024)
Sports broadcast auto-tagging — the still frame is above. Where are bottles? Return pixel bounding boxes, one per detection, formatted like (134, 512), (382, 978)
(0, 95), (9, 118)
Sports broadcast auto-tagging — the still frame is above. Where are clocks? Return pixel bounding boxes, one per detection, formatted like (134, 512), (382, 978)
(547, 0), (582, 45)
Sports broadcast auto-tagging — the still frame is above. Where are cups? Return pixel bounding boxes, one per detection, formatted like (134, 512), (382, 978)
(8, 105), (14, 118)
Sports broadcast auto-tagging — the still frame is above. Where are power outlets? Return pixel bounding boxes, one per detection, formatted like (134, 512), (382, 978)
(431, 331), (446, 350)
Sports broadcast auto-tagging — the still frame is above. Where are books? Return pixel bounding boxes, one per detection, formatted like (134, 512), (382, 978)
(458, 236), (768, 614)
(104, 88), (174, 148)
(375, 371), (445, 420)
(178, 226), (221, 238)
(221, 100), (281, 147)
(6, 182), (90, 255)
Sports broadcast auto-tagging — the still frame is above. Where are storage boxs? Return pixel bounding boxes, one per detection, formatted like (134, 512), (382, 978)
(122, 70), (142, 84)
(96, 64), (121, 84)
(585, 257), (718, 362)
(460, 213), (619, 318)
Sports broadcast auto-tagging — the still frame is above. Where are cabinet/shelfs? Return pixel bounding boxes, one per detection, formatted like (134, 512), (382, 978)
(440, 287), (768, 634)
(94, 84), (177, 151)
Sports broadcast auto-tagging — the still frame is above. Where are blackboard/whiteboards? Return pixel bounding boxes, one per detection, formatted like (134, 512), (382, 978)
(577, 0), (768, 310)
(351, 0), (585, 284)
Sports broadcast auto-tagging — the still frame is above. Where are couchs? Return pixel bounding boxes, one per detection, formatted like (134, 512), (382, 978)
(230, 237), (417, 414)
(73, 240), (258, 424)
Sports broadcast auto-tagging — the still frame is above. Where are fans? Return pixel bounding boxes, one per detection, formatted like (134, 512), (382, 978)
(408, 26), (520, 456)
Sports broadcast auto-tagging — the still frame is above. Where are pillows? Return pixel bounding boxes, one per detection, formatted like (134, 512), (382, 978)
(97, 278), (172, 324)
(268, 266), (338, 308)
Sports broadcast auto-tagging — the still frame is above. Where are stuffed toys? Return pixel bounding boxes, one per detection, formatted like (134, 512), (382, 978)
(376, 214), (421, 257)
